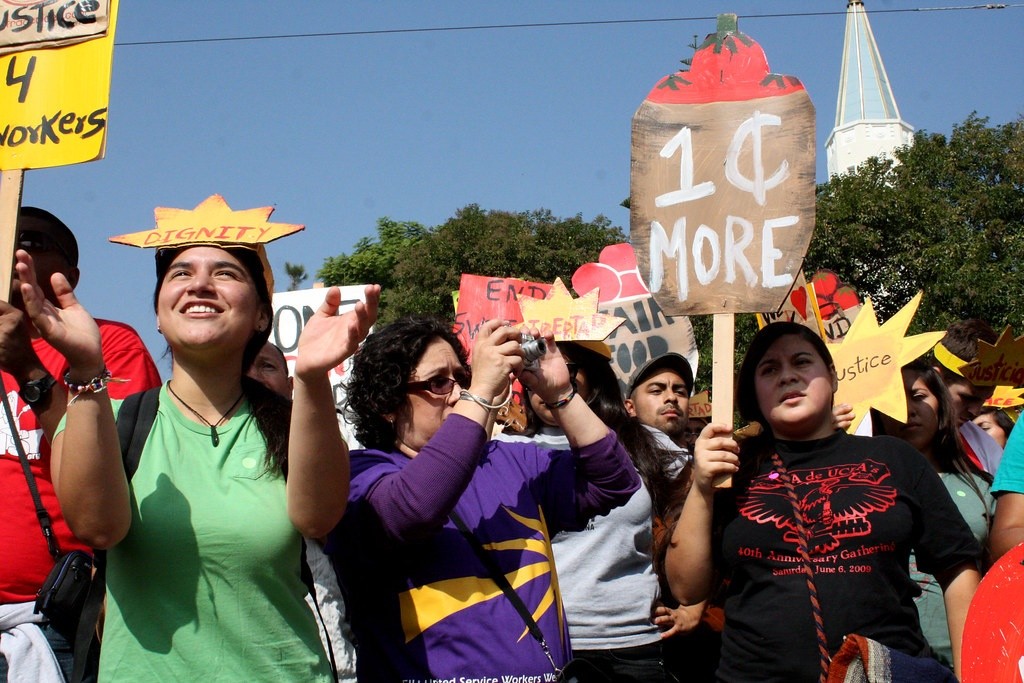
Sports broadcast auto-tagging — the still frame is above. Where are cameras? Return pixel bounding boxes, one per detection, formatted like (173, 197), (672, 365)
(506, 332), (547, 372)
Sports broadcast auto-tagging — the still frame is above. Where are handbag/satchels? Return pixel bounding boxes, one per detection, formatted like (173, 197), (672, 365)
(819, 632), (961, 683)
(37, 548), (90, 623)
(554, 659), (592, 683)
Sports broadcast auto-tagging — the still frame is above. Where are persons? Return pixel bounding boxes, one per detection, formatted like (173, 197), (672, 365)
(248, 339), (359, 683)
(14, 233), (382, 683)
(623, 353), (726, 682)
(490, 341), (708, 683)
(830, 323), (1023, 683)
(0, 207), (161, 683)
(662, 322), (986, 683)
(342, 309), (642, 683)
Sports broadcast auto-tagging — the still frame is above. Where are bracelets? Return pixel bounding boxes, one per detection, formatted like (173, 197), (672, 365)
(545, 380), (577, 410)
(62, 363), (131, 407)
(459, 393), (492, 413)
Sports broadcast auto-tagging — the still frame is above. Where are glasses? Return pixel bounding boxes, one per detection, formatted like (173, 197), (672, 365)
(524, 360), (586, 391)
(16, 228), (74, 263)
(401, 373), (474, 398)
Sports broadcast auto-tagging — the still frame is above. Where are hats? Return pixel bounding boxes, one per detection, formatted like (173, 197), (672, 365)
(625, 352), (694, 399)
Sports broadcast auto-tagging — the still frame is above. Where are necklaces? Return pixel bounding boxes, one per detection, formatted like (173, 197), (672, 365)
(167, 378), (245, 447)
